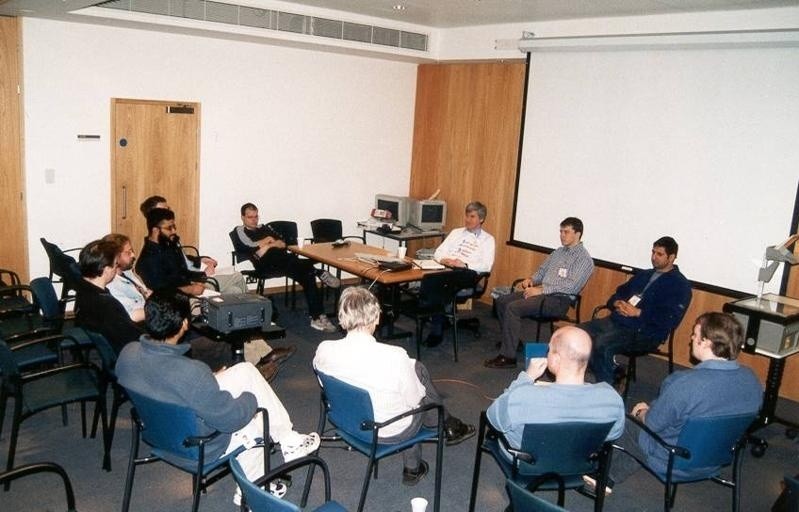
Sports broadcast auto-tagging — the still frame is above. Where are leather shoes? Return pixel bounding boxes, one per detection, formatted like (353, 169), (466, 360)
(259, 344), (298, 383)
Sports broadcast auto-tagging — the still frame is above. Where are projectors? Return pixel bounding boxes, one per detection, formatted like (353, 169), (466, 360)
(208, 293), (273, 334)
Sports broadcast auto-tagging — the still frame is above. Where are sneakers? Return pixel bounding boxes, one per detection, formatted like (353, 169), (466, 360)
(232, 480), (289, 507)
(484, 353), (519, 369)
(494, 337), (525, 353)
(446, 418), (477, 446)
(311, 314), (338, 334)
(582, 469), (616, 497)
(612, 362), (628, 395)
(402, 459), (430, 487)
(282, 432), (324, 465)
(422, 334), (445, 349)
(318, 269), (341, 290)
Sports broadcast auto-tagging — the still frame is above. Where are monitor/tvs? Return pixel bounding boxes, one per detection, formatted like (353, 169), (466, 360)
(409, 198), (447, 232)
(375, 194), (409, 227)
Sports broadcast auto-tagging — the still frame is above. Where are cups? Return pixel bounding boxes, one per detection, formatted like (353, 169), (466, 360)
(398, 247), (407, 258)
(410, 497), (428, 512)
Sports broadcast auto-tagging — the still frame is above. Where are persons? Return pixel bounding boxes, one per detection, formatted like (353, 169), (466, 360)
(137, 194), (248, 295)
(485, 324), (627, 475)
(481, 216), (594, 370)
(134, 209), (210, 312)
(582, 309), (764, 495)
(234, 201), (342, 332)
(96, 233), (203, 341)
(310, 285), (476, 485)
(402, 200), (496, 350)
(113, 282), (322, 505)
(573, 235), (691, 396)
(73, 240), (298, 384)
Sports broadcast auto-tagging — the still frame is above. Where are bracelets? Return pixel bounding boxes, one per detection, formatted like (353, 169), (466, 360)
(520, 280), (527, 291)
(463, 262), (468, 270)
